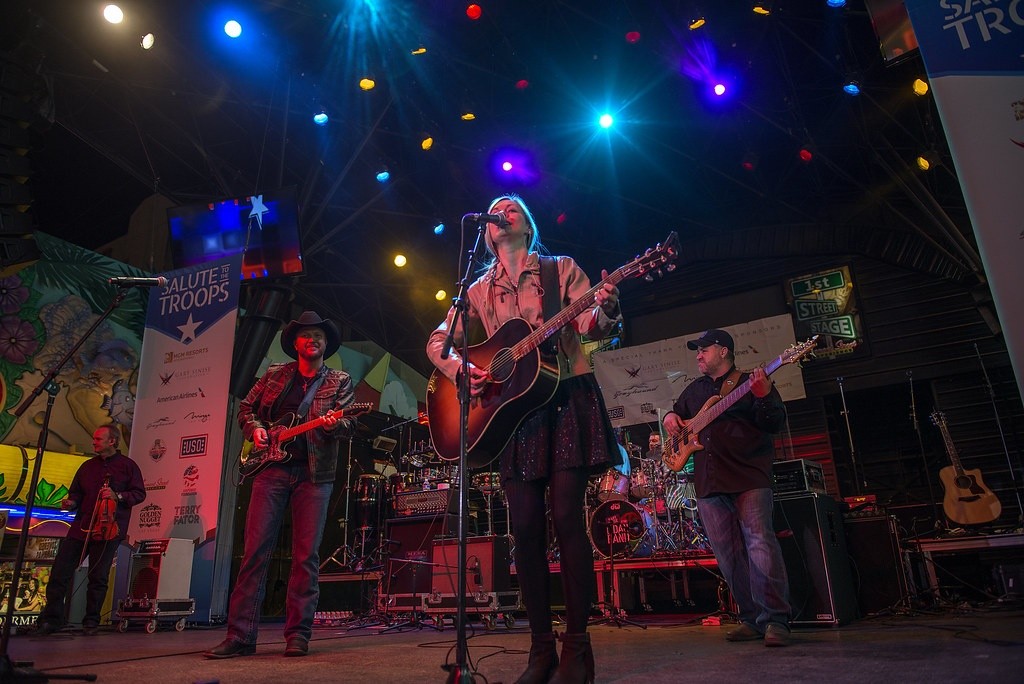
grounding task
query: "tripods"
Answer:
[344,558,474,634]
[318,437,359,570]
[624,462,713,560]
[685,561,738,624]
[586,525,647,630]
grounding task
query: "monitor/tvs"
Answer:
[165,184,307,287]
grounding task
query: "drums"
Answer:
[353,475,387,500]
[630,468,664,498]
[596,471,630,502]
[352,500,379,533]
[590,499,656,558]
[390,471,406,494]
[473,472,501,491]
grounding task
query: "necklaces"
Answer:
[303,374,315,391]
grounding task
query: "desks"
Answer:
[907,532,1024,603]
[604,554,740,621]
[318,571,386,582]
[510,560,606,615]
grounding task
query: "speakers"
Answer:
[772,492,861,625]
[383,513,471,607]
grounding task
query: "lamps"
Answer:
[422,213,446,235]
[751,0,778,16]
[686,5,706,30]
[842,68,867,95]
[917,149,942,171]
[912,74,932,98]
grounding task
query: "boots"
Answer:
[513,631,559,684]
[559,632,596,684]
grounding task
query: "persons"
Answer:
[203,311,357,657]
[662,327,791,645]
[427,196,624,684]
[16,425,146,635]
[649,433,662,449]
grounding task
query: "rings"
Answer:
[604,300,609,306]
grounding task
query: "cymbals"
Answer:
[646,446,662,461]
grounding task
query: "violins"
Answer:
[91,473,120,541]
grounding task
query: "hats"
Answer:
[280,311,342,361]
[686,329,735,351]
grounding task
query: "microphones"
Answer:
[474,557,481,584]
[469,212,506,228]
[108,276,168,287]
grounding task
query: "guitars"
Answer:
[238,402,374,479]
[929,406,1002,525]
[425,230,686,469]
[662,334,819,472]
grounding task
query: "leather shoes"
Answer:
[725,624,765,641]
[765,623,790,647]
[204,637,256,659]
[283,638,308,657]
[38,621,55,636]
[83,621,98,635]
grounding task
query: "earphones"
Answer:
[527,229,533,235]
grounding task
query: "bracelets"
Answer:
[116,491,123,500]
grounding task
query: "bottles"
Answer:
[140,593,149,609]
[423,478,430,490]
[124,593,132,607]
